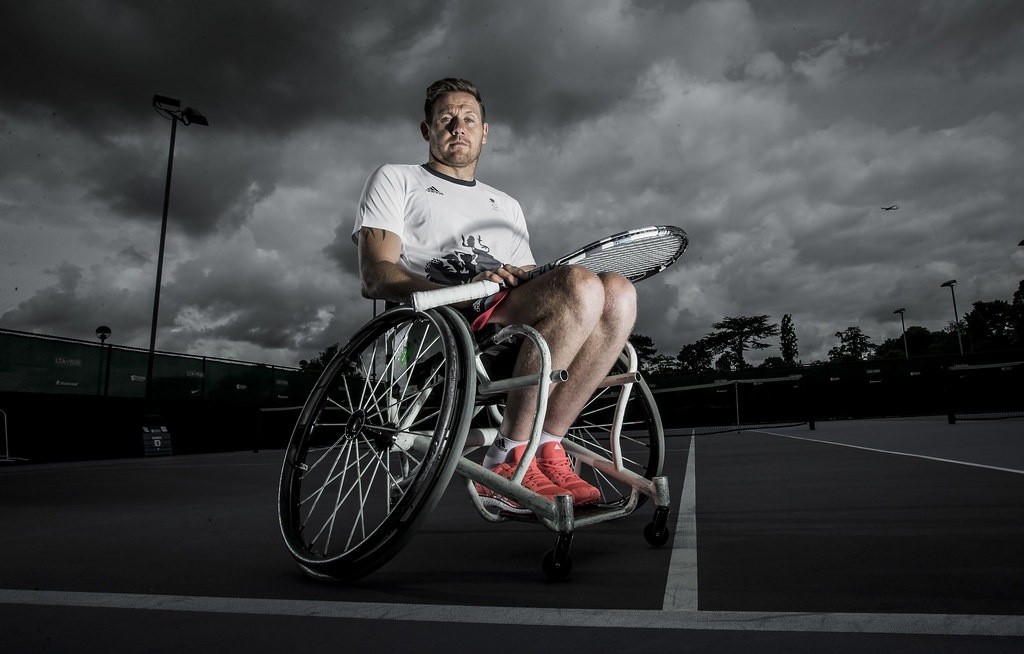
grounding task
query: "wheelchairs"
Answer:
[277,286,673,589]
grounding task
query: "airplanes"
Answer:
[880,204,900,212]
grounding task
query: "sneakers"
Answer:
[475,441,600,514]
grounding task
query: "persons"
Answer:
[351,76,636,515]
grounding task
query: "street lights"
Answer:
[94,322,112,397]
[939,275,972,356]
[140,88,208,416]
[893,304,912,357]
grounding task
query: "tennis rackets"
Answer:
[407,223,688,314]
